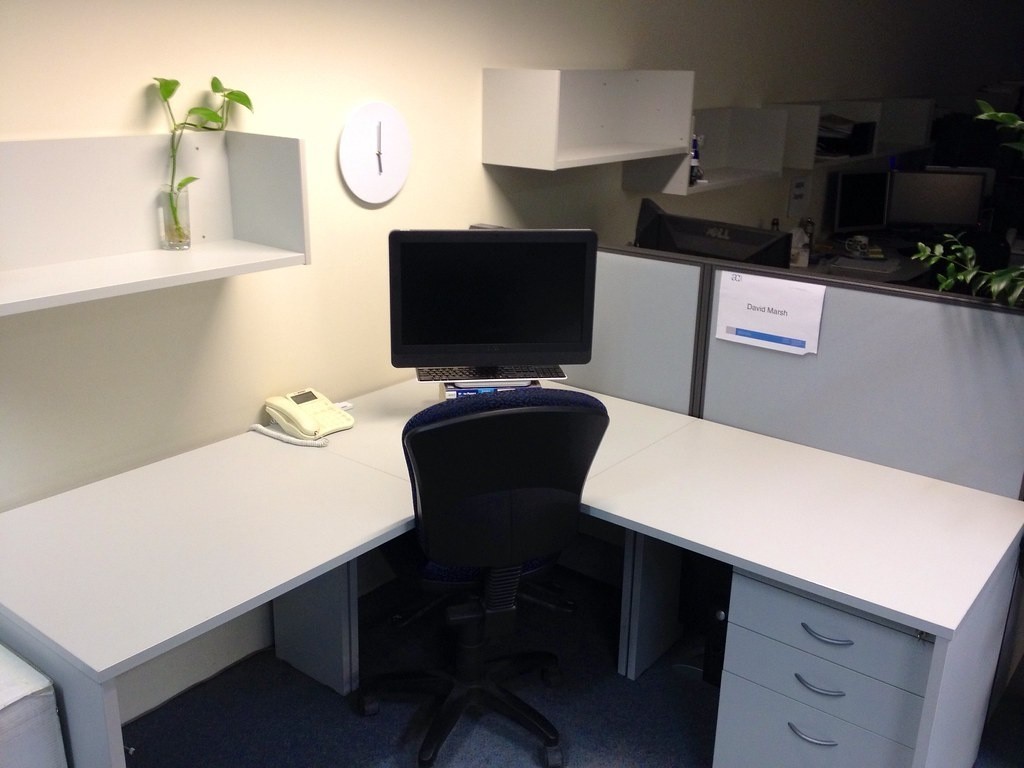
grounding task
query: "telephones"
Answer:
[264,387,354,441]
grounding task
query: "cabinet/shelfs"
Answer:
[481,66,933,195]
[0,130,313,315]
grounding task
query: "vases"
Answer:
[161,187,193,249]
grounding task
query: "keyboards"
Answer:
[416,365,567,381]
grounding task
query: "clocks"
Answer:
[338,100,414,206]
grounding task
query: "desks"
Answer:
[0,379,1024,768]
[809,227,981,284]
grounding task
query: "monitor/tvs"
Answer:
[887,170,985,241]
[828,170,892,235]
[632,197,792,270]
[388,229,597,388]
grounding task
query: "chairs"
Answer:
[930,113,1003,167]
[354,387,608,768]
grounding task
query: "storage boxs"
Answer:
[0,644,68,768]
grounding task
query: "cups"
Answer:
[846,235,868,258]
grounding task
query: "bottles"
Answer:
[689,135,700,185]
[771,219,780,231]
